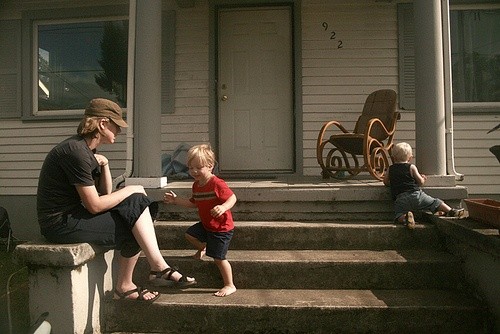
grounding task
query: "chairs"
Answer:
[316,89,401,181]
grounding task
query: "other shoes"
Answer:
[450,208,464,216]
[406,211,415,229]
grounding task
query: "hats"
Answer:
[85,98,128,128]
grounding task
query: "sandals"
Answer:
[148,268,198,287]
[112,288,161,304]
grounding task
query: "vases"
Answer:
[463,198,500,227]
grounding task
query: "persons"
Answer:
[37,99,196,304]
[383,142,464,229]
[164,144,236,297]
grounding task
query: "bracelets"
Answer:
[101,162,109,166]
